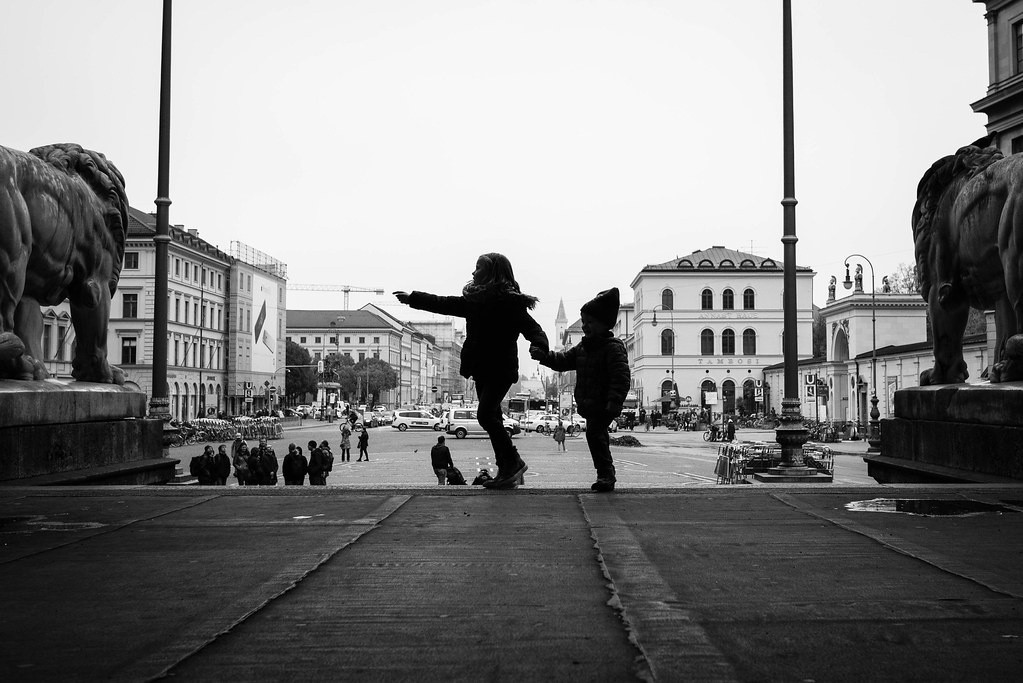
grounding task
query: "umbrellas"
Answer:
[653,395,685,402]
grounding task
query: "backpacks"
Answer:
[315,449,333,472]
[189,454,203,477]
[323,448,334,466]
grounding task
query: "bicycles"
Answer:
[338,417,366,433]
[737,413,840,443]
[703,425,736,443]
[170,415,286,447]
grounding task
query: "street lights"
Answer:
[651,304,676,419]
[842,253,881,452]
[322,321,338,421]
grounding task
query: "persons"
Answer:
[198,445,231,485]
[257,408,279,417]
[553,422,566,451]
[529,287,630,491]
[197,409,204,418]
[303,405,317,419]
[393,253,549,487]
[357,428,369,462]
[346,404,358,432]
[725,419,735,442]
[619,407,720,441]
[282,440,333,485]
[231,433,278,485]
[340,425,352,462]
[431,436,453,485]
[744,407,775,427]
[217,410,226,420]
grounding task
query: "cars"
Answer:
[510,397,618,437]
[410,393,480,418]
[346,404,395,428]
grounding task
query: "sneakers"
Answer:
[496,456,529,487]
[482,470,518,488]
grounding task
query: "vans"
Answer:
[392,410,442,432]
[446,407,522,438]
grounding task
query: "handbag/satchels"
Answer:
[339,442,344,447]
[356,439,361,449]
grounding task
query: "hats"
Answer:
[308,440,317,448]
[235,432,242,439]
[579,286,621,329]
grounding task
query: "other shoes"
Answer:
[356,458,361,461]
[364,458,369,461]
[591,470,617,492]
